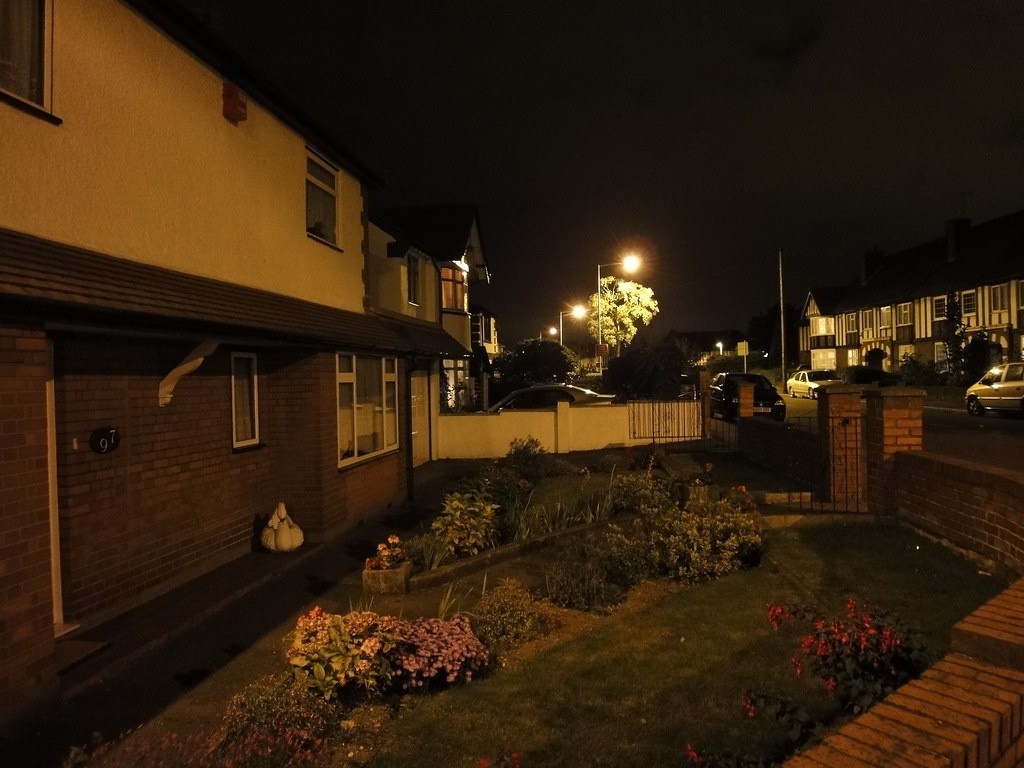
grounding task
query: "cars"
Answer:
[710,372,787,422]
[477,384,618,406]
[795,364,811,372]
[964,362,1024,417]
[788,369,842,400]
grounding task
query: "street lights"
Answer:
[596,256,641,372]
[559,306,587,344]
